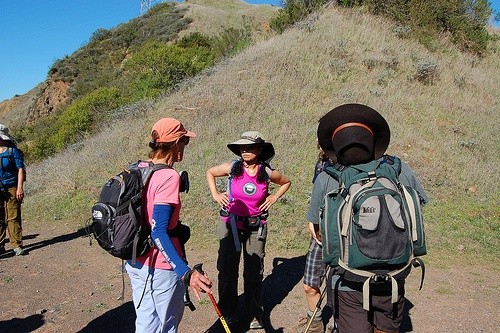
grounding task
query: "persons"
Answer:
[0,124,27,256]
[124,117,213,333]
[307,103,429,333]
[303,142,337,333]
[206,130,291,333]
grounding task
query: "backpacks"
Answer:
[84,159,175,266]
[319,154,427,311]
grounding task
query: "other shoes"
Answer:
[249,317,263,329]
[13,246,25,255]
[219,316,232,329]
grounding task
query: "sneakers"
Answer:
[289,314,325,333]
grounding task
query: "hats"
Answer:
[0,123,13,140]
[227,131,275,161]
[317,103,390,168]
[151,118,196,142]
